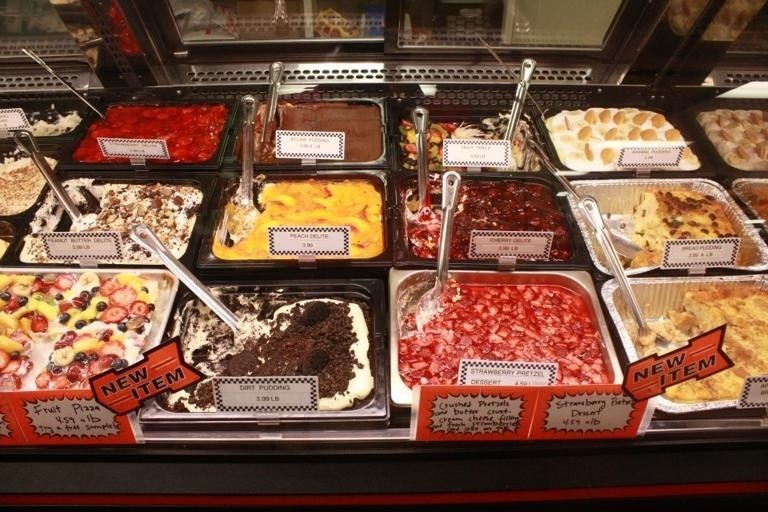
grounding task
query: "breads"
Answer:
[565,110,768,404]
